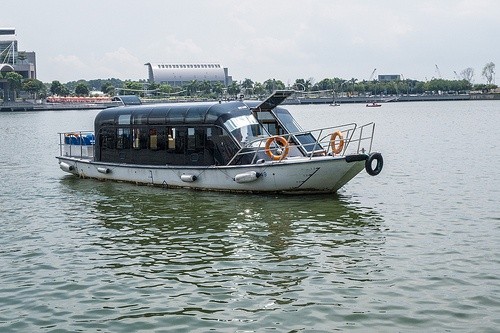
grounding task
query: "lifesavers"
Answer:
[330,132,344,154]
[266,136,289,160]
[365,152,384,176]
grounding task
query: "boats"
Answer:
[366,101,382,107]
[329,101,340,106]
[54,88,384,198]
